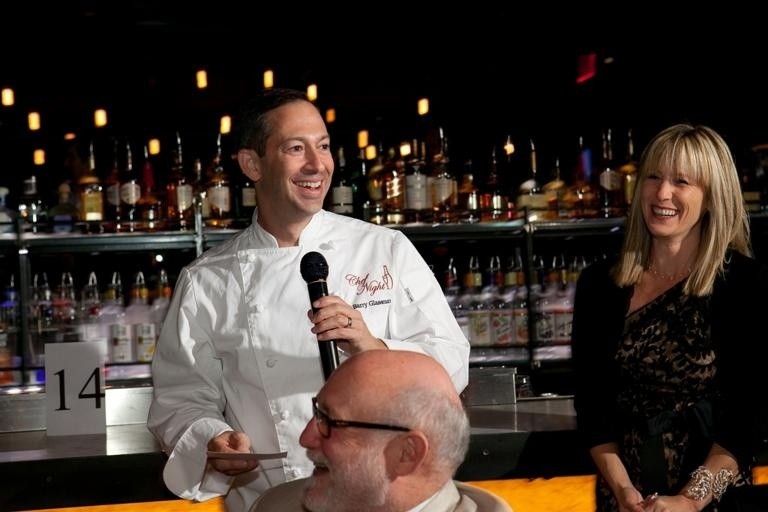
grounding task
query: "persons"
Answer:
[146,87,471,512]
[567,122,767,512]
[247,349,512,512]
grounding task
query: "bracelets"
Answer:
[686,465,734,501]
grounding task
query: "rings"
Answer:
[345,316,353,328]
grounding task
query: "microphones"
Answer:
[300,252,339,382]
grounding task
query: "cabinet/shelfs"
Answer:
[0,209,767,436]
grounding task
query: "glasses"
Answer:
[311,396,411,439]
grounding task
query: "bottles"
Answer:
[0,124,260,235]
[0,268,174,368]
[332,129,640,222]
[437,251,587,348]
